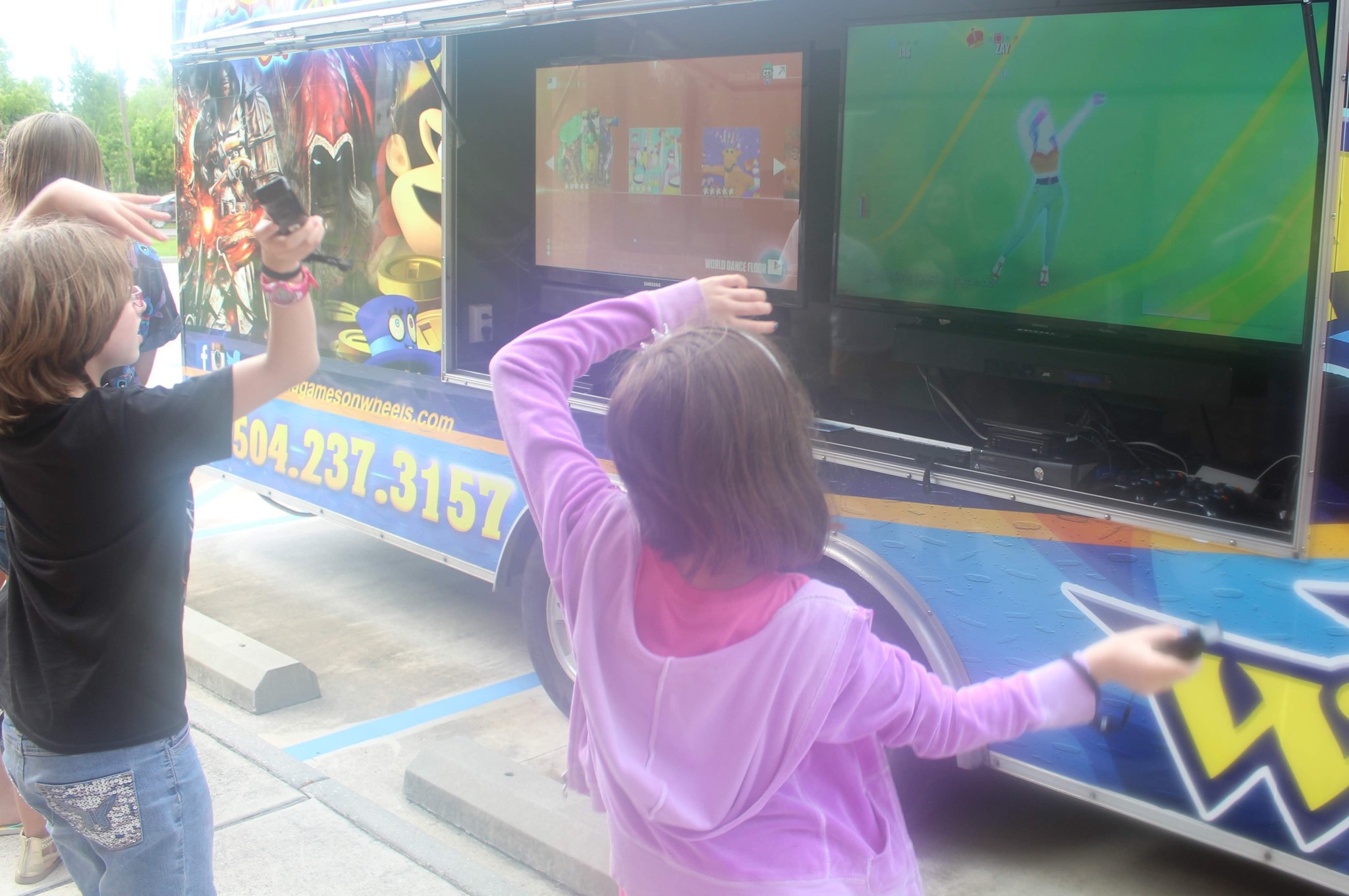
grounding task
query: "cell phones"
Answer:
[1161,624,1220,658]
[255,176,310,237]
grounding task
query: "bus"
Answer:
[172,1,1349,896]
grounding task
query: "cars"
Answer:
[138,193,176,229]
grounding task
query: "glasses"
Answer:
[126,285,143,307]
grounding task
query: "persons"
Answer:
[489,275,1200,896]
[0,176,325,896]
[0,113,181,883]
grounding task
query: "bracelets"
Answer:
[260,261,302,280]
[1063,652,1122,732]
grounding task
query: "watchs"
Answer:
[258,266,319,306]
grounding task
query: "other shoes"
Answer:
[0,822,23,836]
[16,828,62,885]
